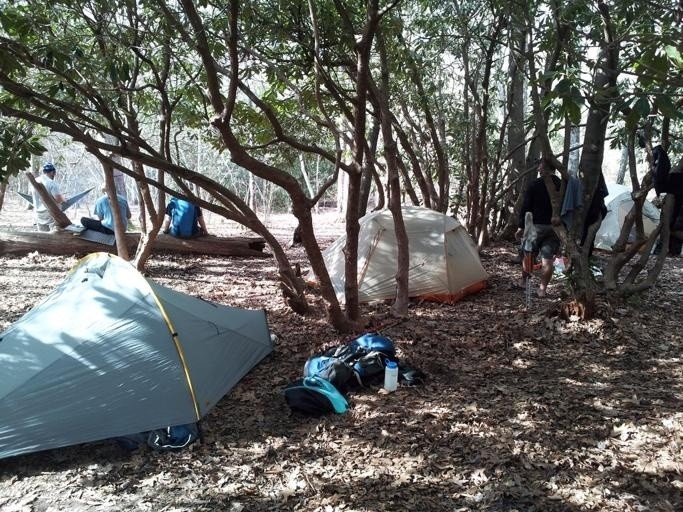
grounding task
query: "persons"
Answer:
[26,161,65,233]
[161,188,210,240]
[79,180,132,236]
[514,156,564,299]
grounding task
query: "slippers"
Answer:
[510,281,527,291]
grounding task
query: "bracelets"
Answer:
[162,230,169,234]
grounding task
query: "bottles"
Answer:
[384,357,398,392]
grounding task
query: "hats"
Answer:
[42,162,54,171]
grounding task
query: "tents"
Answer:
[0,251,275,461]
[304,203,492,307]
[591,184,662,255]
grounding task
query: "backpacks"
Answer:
[302,332,396,390]
